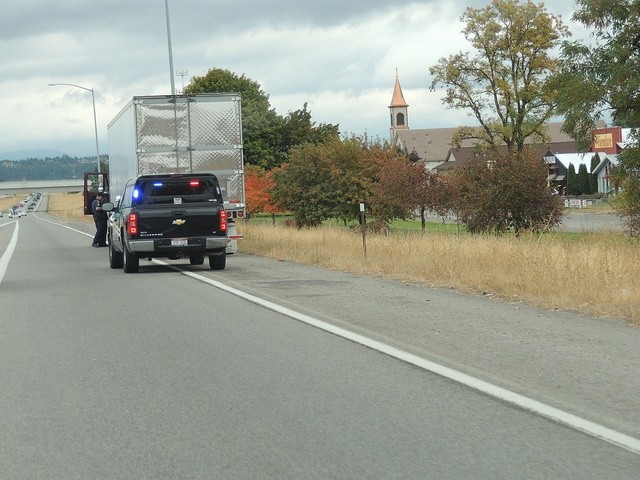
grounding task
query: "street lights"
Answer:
[46,80,105,183]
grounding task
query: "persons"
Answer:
[90,193,110,249]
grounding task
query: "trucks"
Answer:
[83,91,246,254]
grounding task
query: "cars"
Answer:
[0,192,41,218]
[99,172,228,273]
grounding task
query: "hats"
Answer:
[98,192,106,196]
